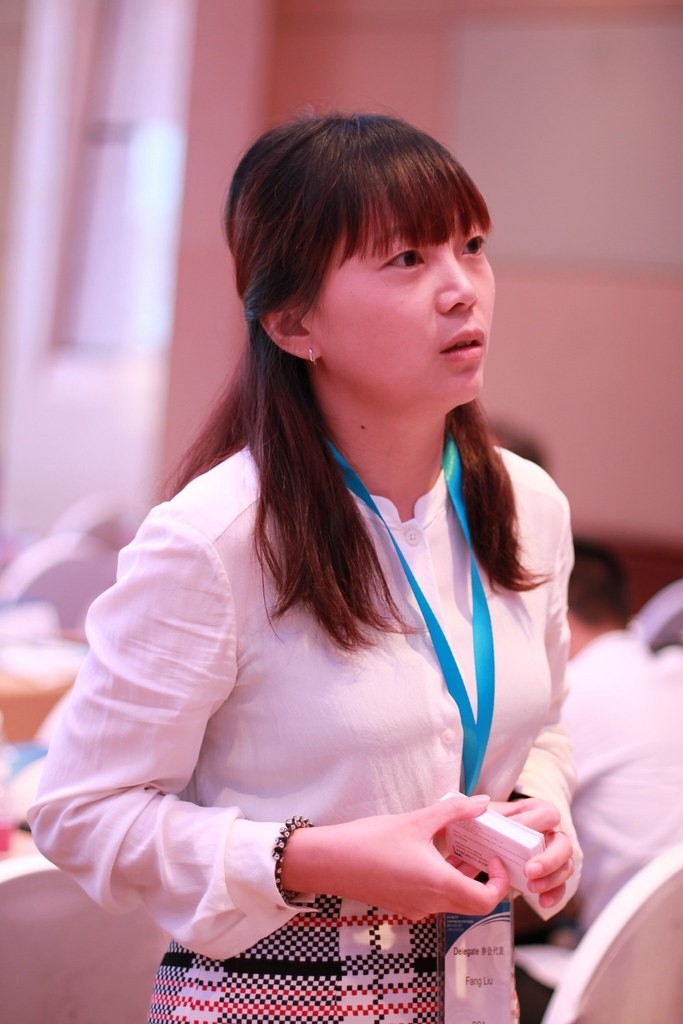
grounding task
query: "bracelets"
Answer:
[273,816,314,902]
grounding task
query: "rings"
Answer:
[571,857,574,864]
[547,831,567,841]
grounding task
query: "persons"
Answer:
[29,114,586,1023]
[514,538,682,1023]
